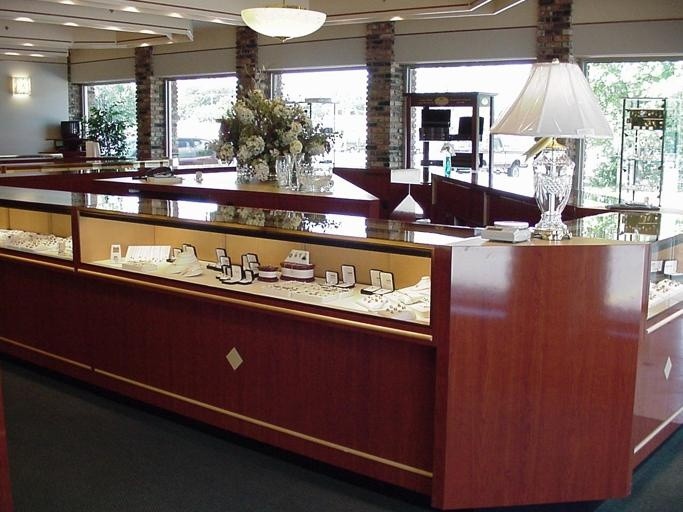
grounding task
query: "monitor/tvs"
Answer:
[61,121,80,137]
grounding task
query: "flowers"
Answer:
[216,64,344,183]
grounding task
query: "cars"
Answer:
[175,137,218,166]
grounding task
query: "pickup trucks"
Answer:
[451,133,529,179]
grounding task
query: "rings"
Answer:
[9,230,57,249]
[649,279,680,301]
[273,280,405,316]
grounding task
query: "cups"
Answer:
[273,150,334,194]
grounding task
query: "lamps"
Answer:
[488,61,614,243]
[8,76,33,95]
[240,0,327,44]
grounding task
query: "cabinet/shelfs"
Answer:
[429,173,577,227]
[405,91,498,227]
[37,138,94,155]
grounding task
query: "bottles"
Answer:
[443,147,451,175]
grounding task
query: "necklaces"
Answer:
[128,245,165,263]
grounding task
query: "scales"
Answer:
[480,221,531,243]
